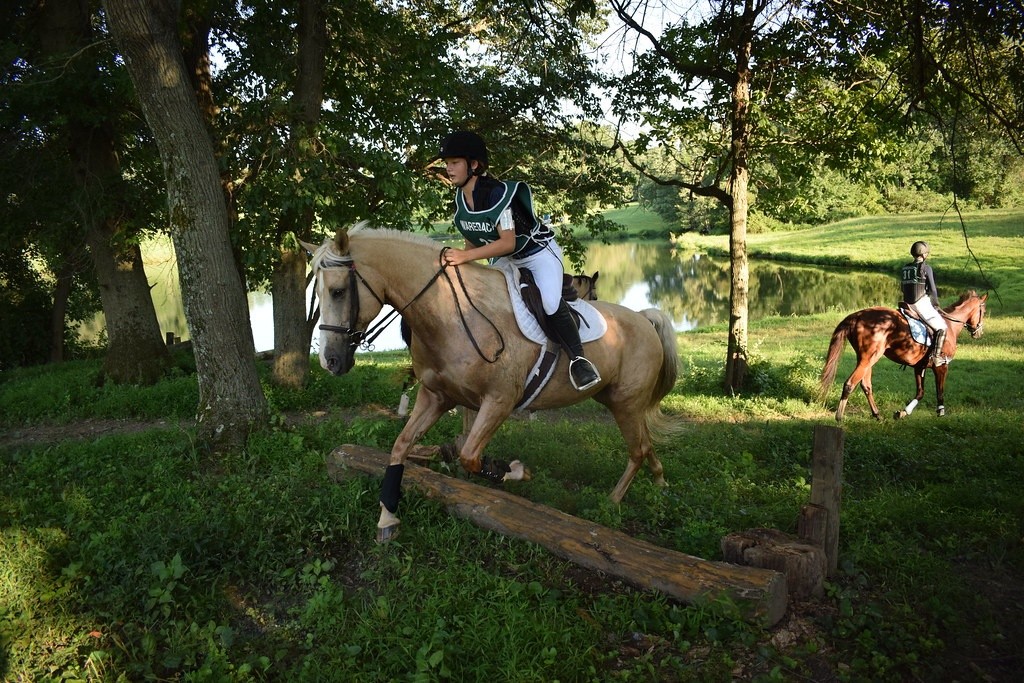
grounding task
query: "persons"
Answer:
[901,241,947,367]
[440,131,598,388]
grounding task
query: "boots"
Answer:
[546,297,596,387]
[933,329,952,367]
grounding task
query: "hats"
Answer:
[432,131,488,169]
[911,241,929,255]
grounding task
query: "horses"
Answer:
[296,222,678,545]
[820,289,989,422]
